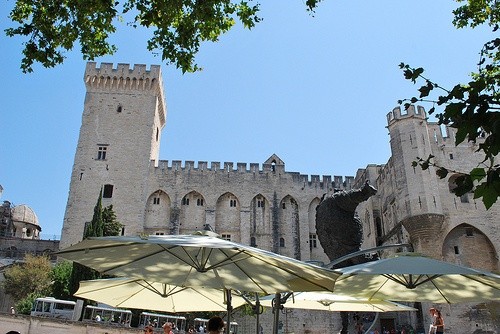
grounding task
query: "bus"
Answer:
[190,318,238,334]
[81,304,132,328]
[139,312,188,334]
[32,297,77,319]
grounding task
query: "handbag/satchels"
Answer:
[428,325,437,334]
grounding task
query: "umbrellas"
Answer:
[53,229,342,334]
[240,290,419,311]
[257,257,500,303]
[73,277,250,313]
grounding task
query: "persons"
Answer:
[428,307,438,334]
[92,314,226,334]
[356,323,425,334]
[434,310,445,334]
